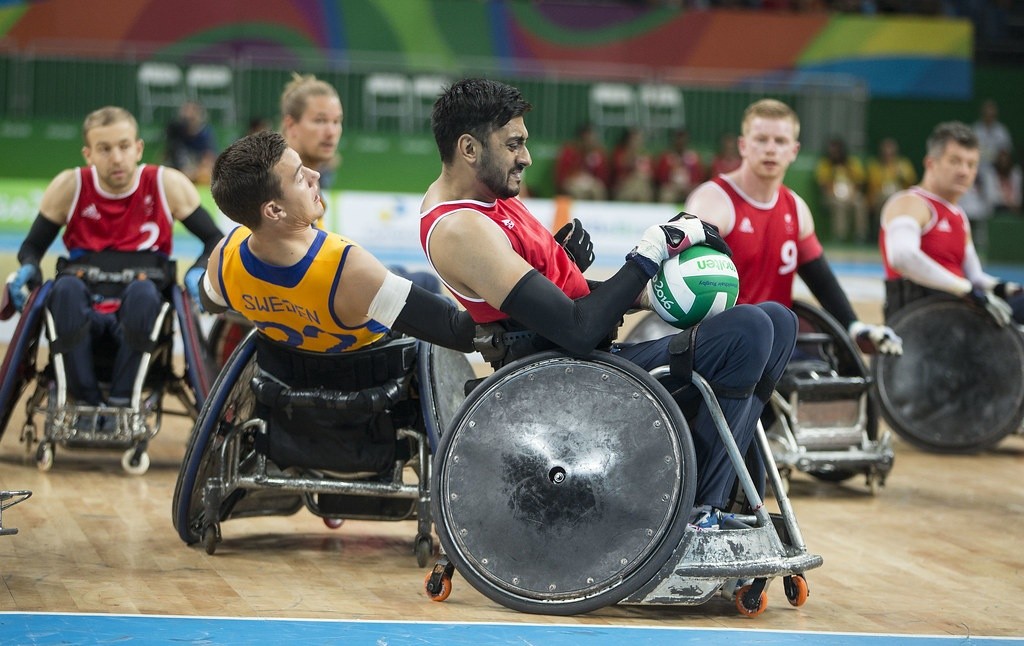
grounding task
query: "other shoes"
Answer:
[693,509,725,530]
[74,398,130,432]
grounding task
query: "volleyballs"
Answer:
[644,245,741,330]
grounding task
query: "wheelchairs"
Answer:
[0,277,211,478]
[428,347,823,618]
[175,325,478,569]
[872,287,1024,452]
[766,297,905,498]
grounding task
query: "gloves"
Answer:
[8,257,43,313]
[995,281,1020,301]
[185,256,214,315]
[553,218,595,273]
[626,212,733,278]
[849,321,903,357]
[967,283,1012,328]
[640,287,654,311]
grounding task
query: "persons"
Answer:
[417,77,801,533]
[167,103,215,193]
[558,98,1023,245]
[683,99,907,357]
[199,127,597,356]
[876,122,1023,331]
[272,72,448,301]
[9,103,229,408]
